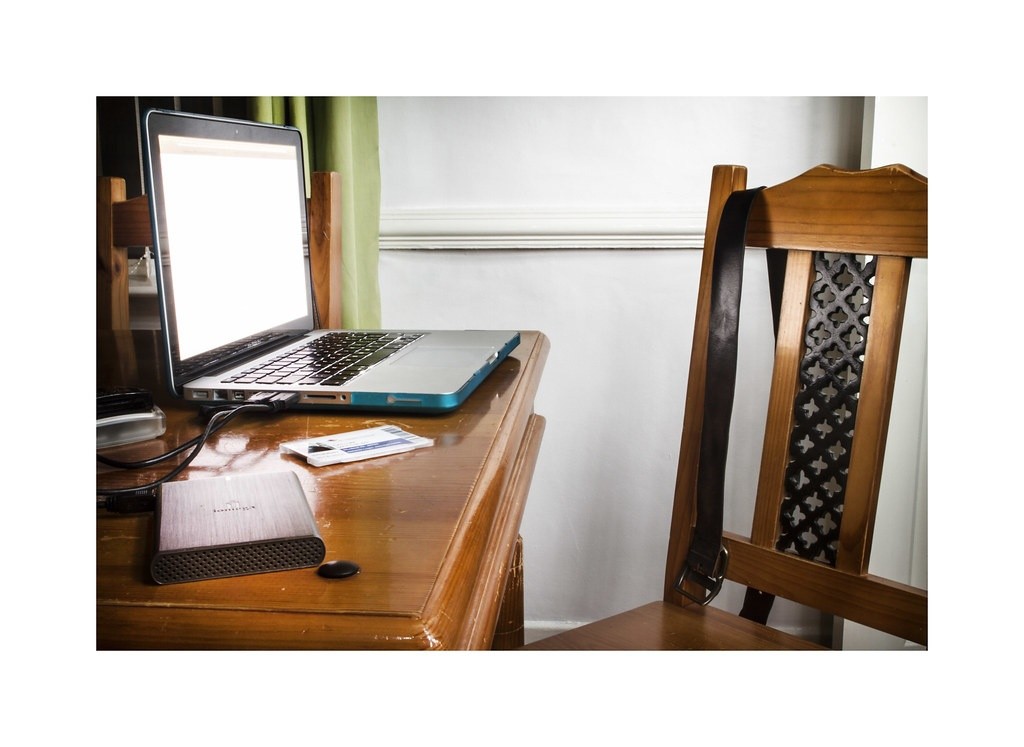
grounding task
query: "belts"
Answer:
[668,183,789,626]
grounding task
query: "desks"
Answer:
[96,329,551,651]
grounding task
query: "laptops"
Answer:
[142,109,521,412]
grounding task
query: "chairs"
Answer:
[96,171,342,330]
[507,163,928,651]
